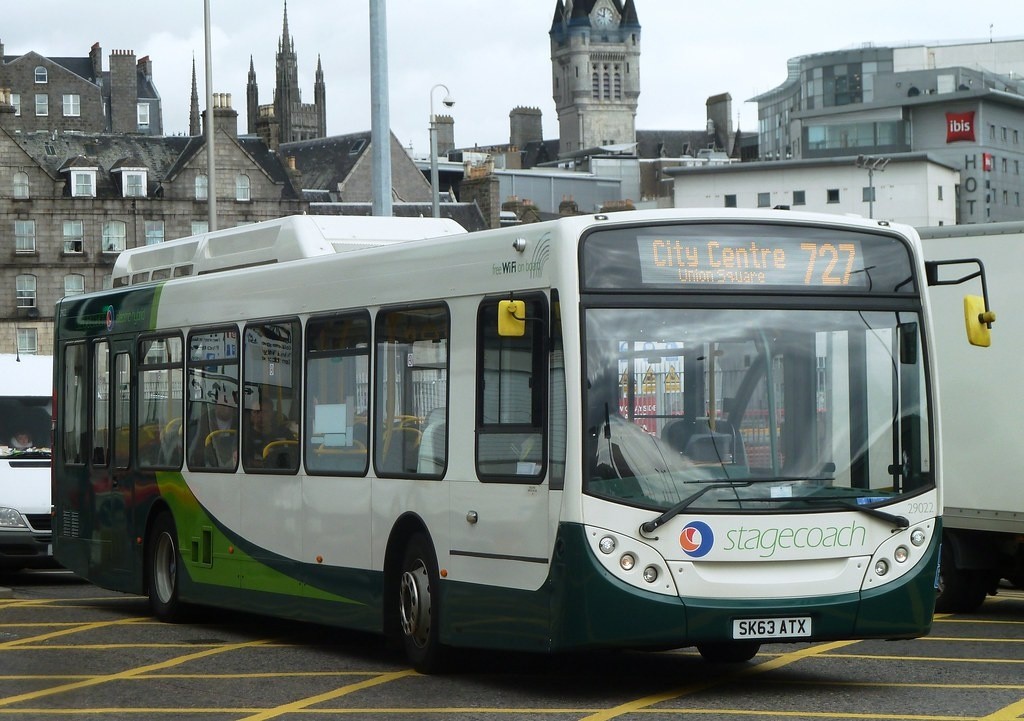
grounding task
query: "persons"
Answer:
[183,386,302,470]
[8,431,33,451]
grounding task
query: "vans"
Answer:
[0,353,79,579]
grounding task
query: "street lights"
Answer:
[429,82,456,217]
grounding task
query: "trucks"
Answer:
[827,219,1024,611]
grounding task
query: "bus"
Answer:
[49,206,996,683]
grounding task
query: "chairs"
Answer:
[660,417,752,484]
[75,414,424,471]
[416,419,445,479]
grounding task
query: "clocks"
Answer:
[595,7,615,29]
